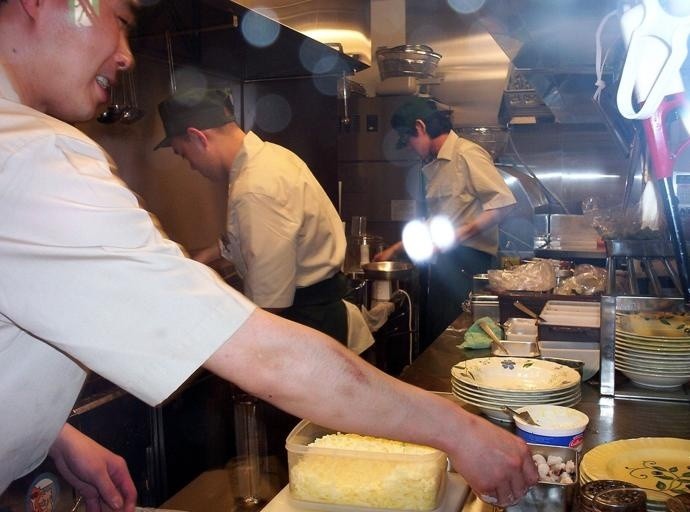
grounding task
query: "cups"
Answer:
[511,404,590,463]
[349,215,368,237]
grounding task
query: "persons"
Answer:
[151,84,377,357]
[0,0,538,512]
[373,99,517,339]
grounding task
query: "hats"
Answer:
[391,98,455,150]
[153,87,238,151]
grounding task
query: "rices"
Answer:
[290,431,449,512]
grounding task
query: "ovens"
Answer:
[336,90,453,247]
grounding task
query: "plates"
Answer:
[614,311,690,391]
[450,357,584,424]
[578,436,690,512]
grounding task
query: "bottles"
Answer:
[360,238,371,269]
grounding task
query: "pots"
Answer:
[360,261,415,281]
[374,76,445,95]
